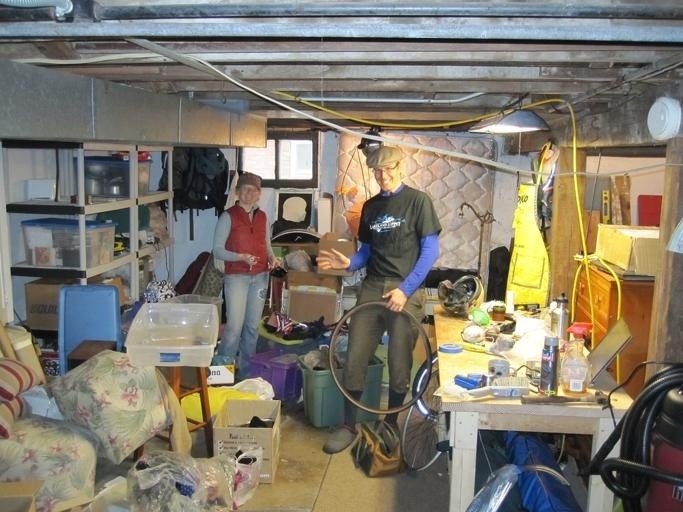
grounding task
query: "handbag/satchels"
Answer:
[353,421,402,477]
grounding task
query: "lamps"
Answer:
[495,110,550,134]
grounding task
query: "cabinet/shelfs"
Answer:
[573,256,655,401]
[0,141,174,339]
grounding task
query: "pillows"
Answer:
[0,356,43,438]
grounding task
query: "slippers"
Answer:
[323,425,358,453]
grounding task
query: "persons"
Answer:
[315,147,442,453]
[211,172,282,379]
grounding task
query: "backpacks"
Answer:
[155,146,228,210]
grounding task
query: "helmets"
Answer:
[438,275,484,317]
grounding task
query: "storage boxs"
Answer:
[596,223,658,275]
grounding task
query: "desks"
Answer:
[434,304,633,512]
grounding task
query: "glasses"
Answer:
[372,162,399,174]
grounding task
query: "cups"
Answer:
[487,359,517,386]
[493,305,506,321]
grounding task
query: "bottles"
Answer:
[540,334,560,396]
[560,339,591,397]
[551,292,572,343]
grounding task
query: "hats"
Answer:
[367,146,403,168]
[237,174,261,191]
